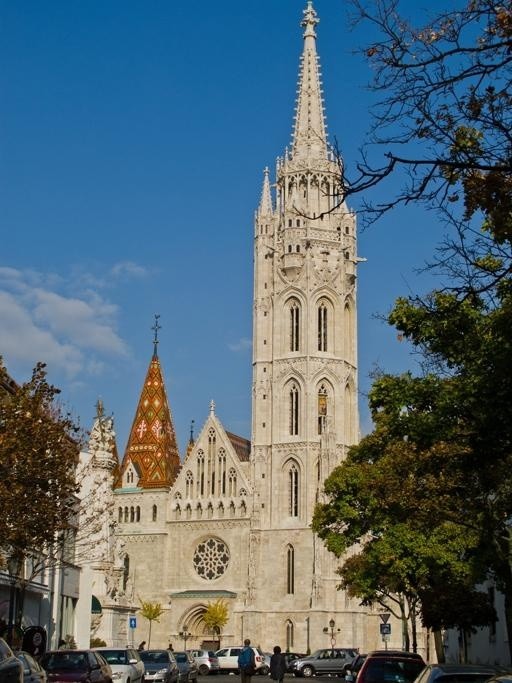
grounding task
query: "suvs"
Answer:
[289,648,361,678]
[215,645,266,675]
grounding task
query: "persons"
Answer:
[139,639,145,650]
[238,639,255,682]
[165,643,174,650]
[270,645,286,682]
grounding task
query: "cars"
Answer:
[282,653,310,672]
[172,651,199,683]
[414,665,498,683]
[346,654,367,683]
[485,673,511,683]
[89,648,145,683]
[188,649,222,676]
[38,650,114,682]
[1,638,25,683]
[261,651,274,674]
[346,650,428,683]
[140,649,179,683]
[13,650,48,683]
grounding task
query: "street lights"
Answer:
[6,548,25,646]
[323,618,341,649]
[179,625,194,650]
